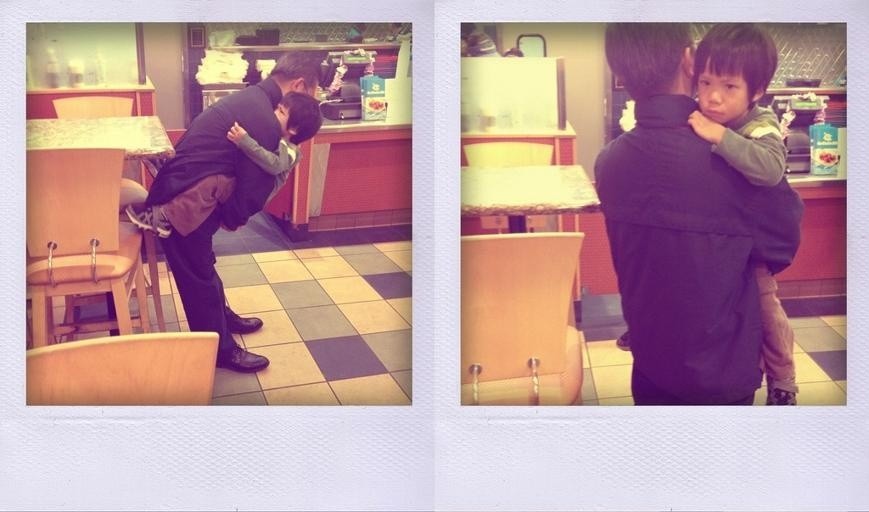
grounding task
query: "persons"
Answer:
[144,51,323,373]
[123,92,322,239]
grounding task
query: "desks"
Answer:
[461,158,603,238]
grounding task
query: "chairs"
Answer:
[461,140,587,406]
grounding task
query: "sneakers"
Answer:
[616,330,633,352]
[125,202,173,239]
[765,386,797,405]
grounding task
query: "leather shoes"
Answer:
[226,305,263,333]
[216,344,270,373]
